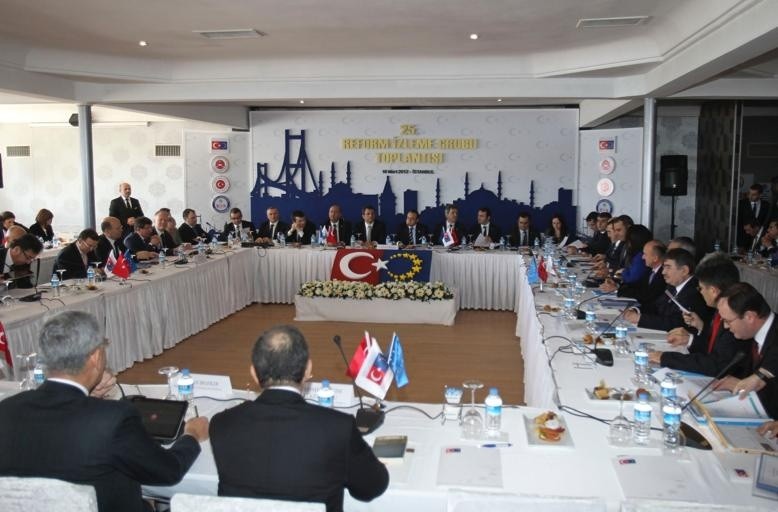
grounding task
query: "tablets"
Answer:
[131,396,188,441]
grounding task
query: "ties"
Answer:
[160,235,165,248]
[236,225,526,245]
[751,340,759,370]
[708,313,720,351]
[126,199,129,208]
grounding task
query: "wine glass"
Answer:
[461,377,484,438]
[17,352,37,391]
[1,234,221,311]
[158,366,178,401]
[608,386,632,446]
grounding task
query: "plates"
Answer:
[525,413,574,449]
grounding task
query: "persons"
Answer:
[86,208,216,268]
[220,208,505,250]
[109,182,142,244]
[579,212,778,440]
[208,324,389,512]
[736,183,770,252]
[0,207,55,293]
[1,310,210,510]
[510,212,578,249]
[50,228,100,284]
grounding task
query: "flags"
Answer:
[345,333,408,401]
[103,247,139,286]
[331,249,433,286]
[527,255,550,291]
[0,323,14,367]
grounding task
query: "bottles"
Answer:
[35,357,47,387]
[239,234,514,252]
[317,379,334,410]
[484,387,503,434]
[442,382,465,429]
[179,367,194,421]
[714,238,772,269]
[530,238,690,452]
[50,232,234,297]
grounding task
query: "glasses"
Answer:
[22,250,34,263]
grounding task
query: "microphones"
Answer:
[206,221,220,232]
[681,351,746,450]
[333,335,384,435]
[594,301,633,365]
[18,258,41,302]
[575,284,626,318]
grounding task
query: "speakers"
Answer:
[660,155,688,196]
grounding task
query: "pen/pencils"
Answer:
[194,406,199,417]
